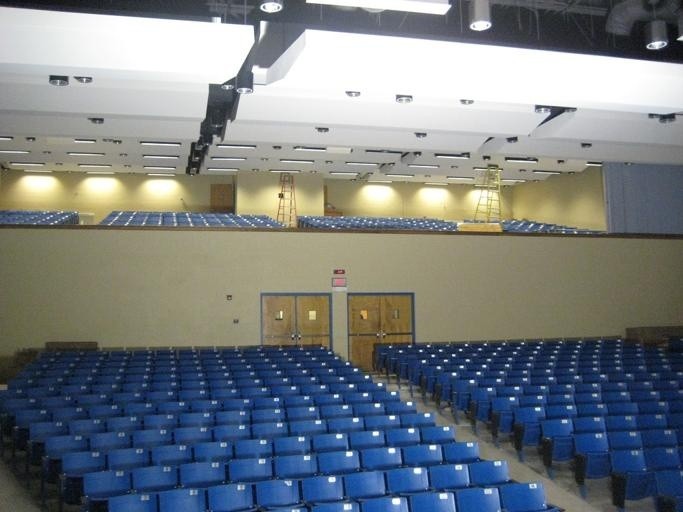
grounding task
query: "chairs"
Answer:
[99,212,288,229]
[299,214,458,232]
[1,211,81,228]
[370,339,682,507]
[4,341,563,510]
[464,217,609,237]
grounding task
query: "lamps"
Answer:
[644,0,683,51]
[395,95,413,104]
[185,0,285,176]
[660,113,676,124]
[535,105,552,114]
[468,0,492,31]
[49,76,69,88]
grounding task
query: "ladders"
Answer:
[474,164,502,226]
[276,175,297,226]
